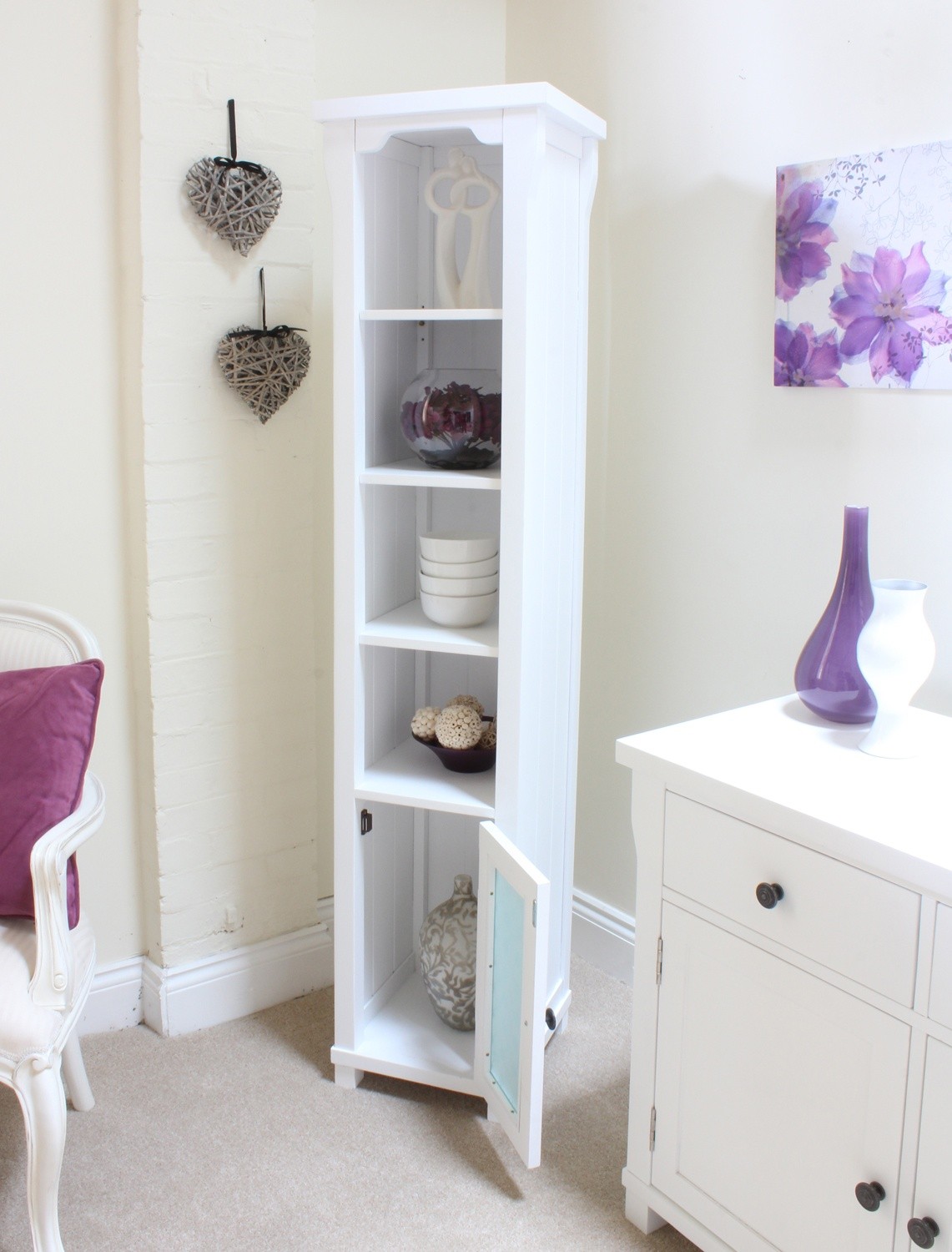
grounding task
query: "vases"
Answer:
[857,578,934,759]
[794,504,877,724]
[420,874,477,1031]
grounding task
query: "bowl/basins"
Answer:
[417,529,500,628]
[410,715,496,772]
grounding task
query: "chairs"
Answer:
[0,597,107,1252]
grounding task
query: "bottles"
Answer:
[398,367,501,469]
[419,874,476,1031]
[856,579,936,759]
[794,502,878,723]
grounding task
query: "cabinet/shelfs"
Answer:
[616,690,952,1252]
[312,83,607,1170]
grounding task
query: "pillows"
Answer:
[0,659,105,930]
